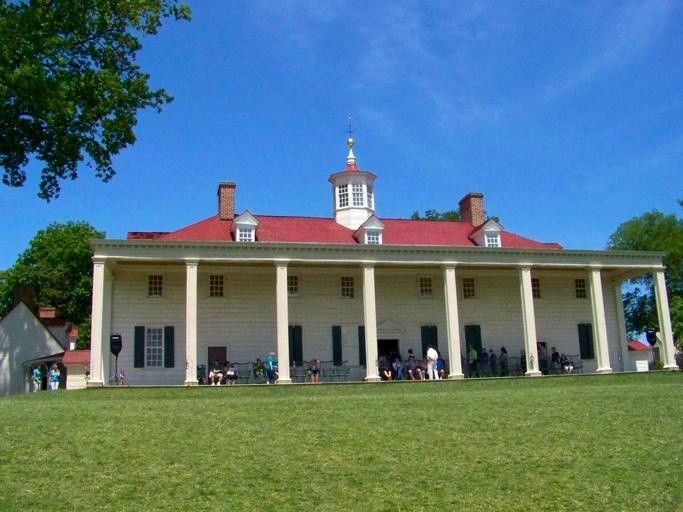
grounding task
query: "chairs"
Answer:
[204,358,351,385]
[377,350,585,382]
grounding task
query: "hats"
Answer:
[268,351,275,355]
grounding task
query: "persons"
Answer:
[262,350,278,384]
[30,366,42,392]
[253,354,264,383]
[224,361,238,385]
[209,359,224,386]
[309,359,319,383]
[119,367,125,385]
[378,342,575,381]
[46,363,61,390]
[288,360,297,384]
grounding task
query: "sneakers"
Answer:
[469,371,573,378]
[211,382,236,385]
[382,376,443,381]
